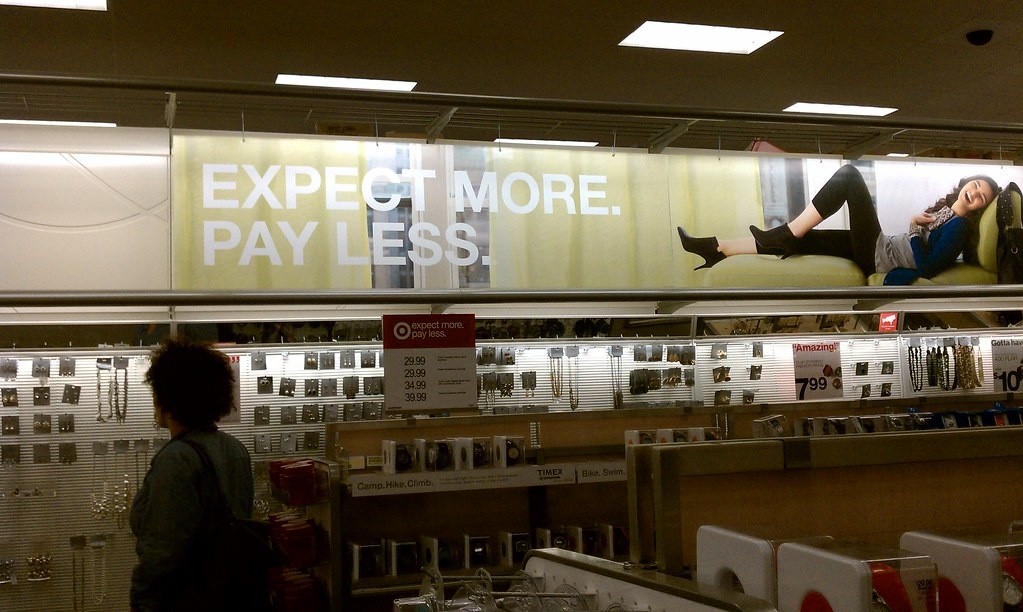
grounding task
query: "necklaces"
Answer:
[549,357,564,397]
[95,369,113,424]
[908,346,923,391]
[91,454,109,518]
[609,355,624,409]
[957,344,985,389]
[926,346,938,387]
[135,450,148,495]
[113,452,130,530]
[115,368,129,424]
[568,356,580,411]
[72,550,84,612]
[90,546,106,604]
[936,346,958,391]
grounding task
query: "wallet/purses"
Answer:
[925,205,954,232]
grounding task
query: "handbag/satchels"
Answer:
[996,182,1023,284]
[175,437,274,612]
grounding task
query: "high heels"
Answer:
[749,222,804,259]
[678,226,727,271]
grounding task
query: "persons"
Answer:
[126,337,254,612]
[676,163,998,279]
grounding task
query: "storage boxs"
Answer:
[493,435,526,467]
[348,538,386,579]
[1008,408,1023,425]
[751,414,792,437]
[388,522,631,612]
[625,430,657,448]
[416,438,458,474]
[690,427,722,440]
[820,416,850,434]
[935,411,963,428]
[906,411,938,430]
[457,436,492,470]
[877,413,914,432]
[959,411,988,427]
[986,408,1014,426]
[850,414,883,433]
[657,428,690,443]
[800,416,829,435]
[381,439,418,476]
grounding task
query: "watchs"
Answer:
[705,430,717,441]
[396,445,413,472]
[673,432,686,442]
[506,440,521,464]
[357,540,491,579]
[551,532,567,549]
[639,432,652,444]
[944,413,1023,430]
[473,442,485,468]
[767,418,877,436]
[513,535,532,563]
[437,442,451,470]
[889,416,905,431]
[916,415,930,430]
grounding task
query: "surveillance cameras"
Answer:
[966,29,994,47]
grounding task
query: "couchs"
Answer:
[704,188,1021,289]
